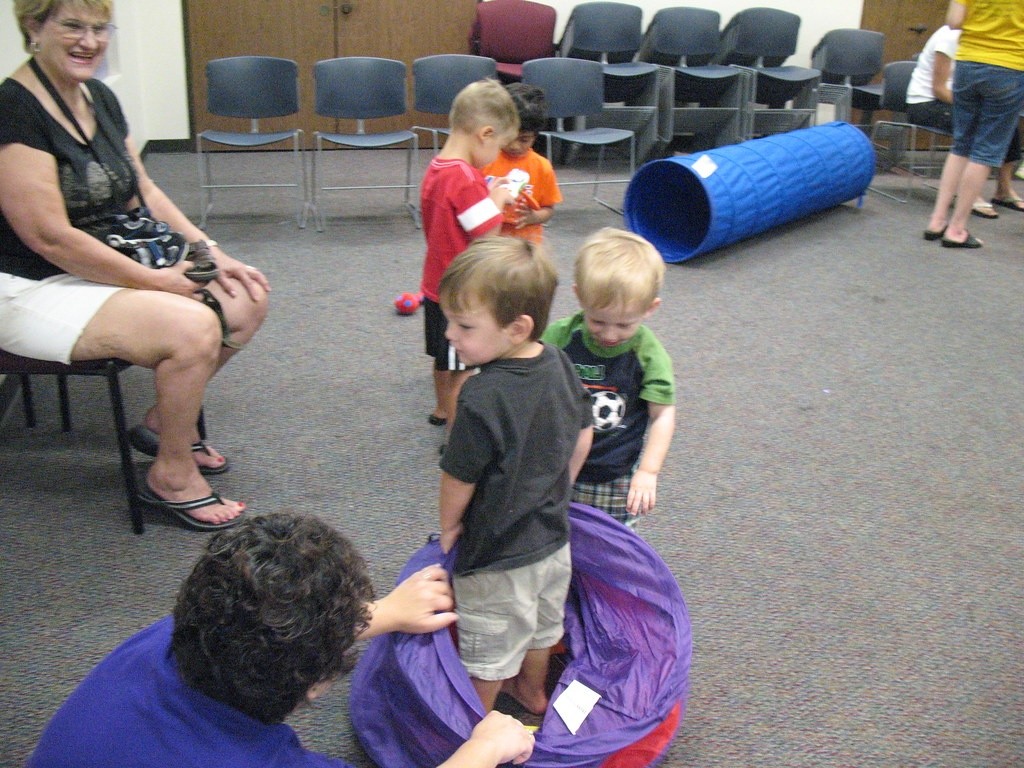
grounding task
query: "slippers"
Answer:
[127,425,228,473]
[991,197,1024,211]
[428,413,449,425]
[952,199,998,217]
[134,478,244,528]
[925,224,950,240]
[941,229,983,248]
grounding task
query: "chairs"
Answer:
[311,56,423,232]
[197,54,308,231]
[413,54,497,213]
[470,0,955,227]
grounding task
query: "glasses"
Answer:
[45,17,119,42]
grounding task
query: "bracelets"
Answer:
[206,240,219,247]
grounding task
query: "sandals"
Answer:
[186,239,218,279]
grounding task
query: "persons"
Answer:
[420,78,524,453]
[26,511,535,768]
[538,228,678,531]
[0,0,272,531]
[906,24,1024,219]
[478,83,563,244]
[924,0,1024,249]
[438,237,595,718]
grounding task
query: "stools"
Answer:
[0,347,206,534]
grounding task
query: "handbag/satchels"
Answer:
[107,214,186,272]
